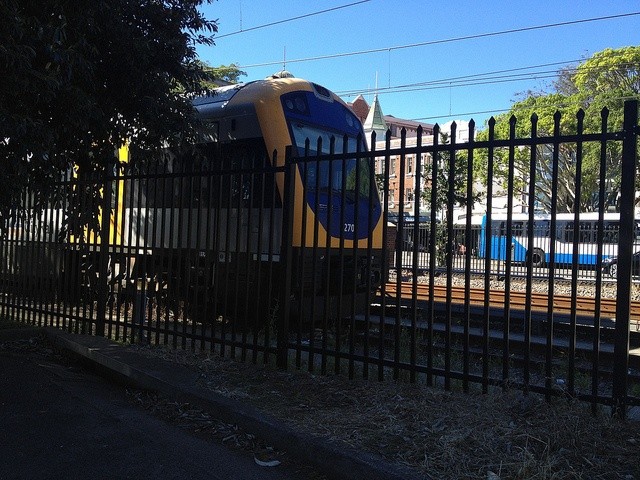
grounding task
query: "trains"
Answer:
[0,78,383,326]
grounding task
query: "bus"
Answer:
[479,214,640,278]
